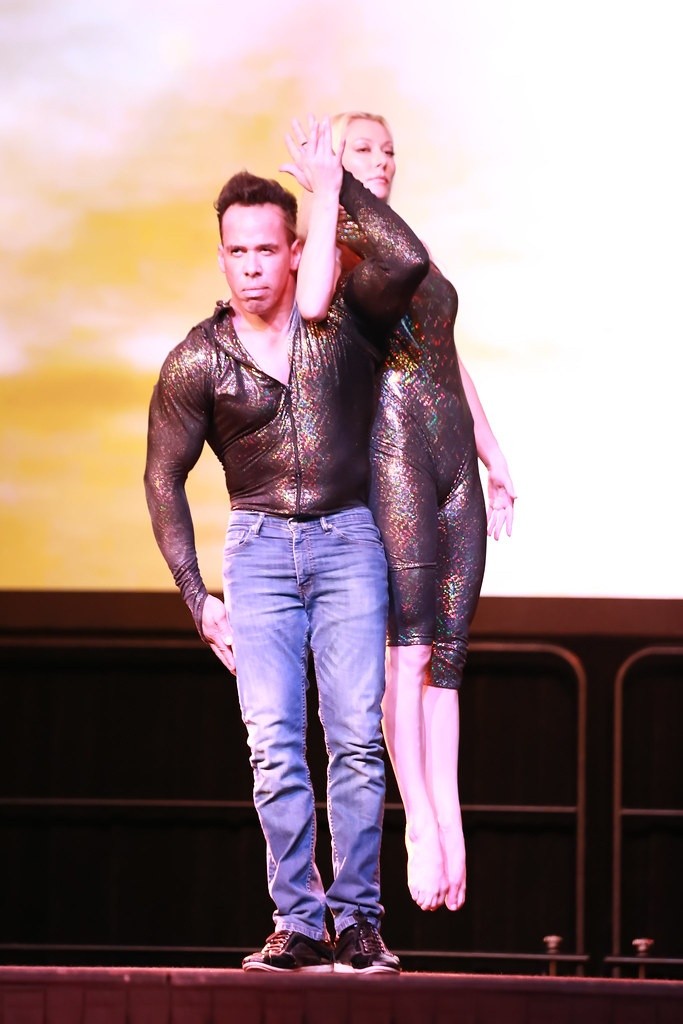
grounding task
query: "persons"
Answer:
[144,113,432,975]
[294,112,518,910]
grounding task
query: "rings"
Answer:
[302,142,307,146]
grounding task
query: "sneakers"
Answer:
[242,930,335,973]
[334,902,402,974]
